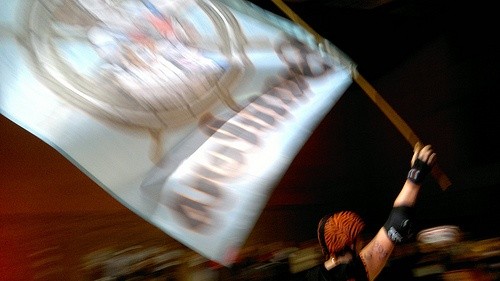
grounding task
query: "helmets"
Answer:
[319,209,363,257]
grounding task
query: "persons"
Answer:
[300,145,437,281]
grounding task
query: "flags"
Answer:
[0,0,354,268]
